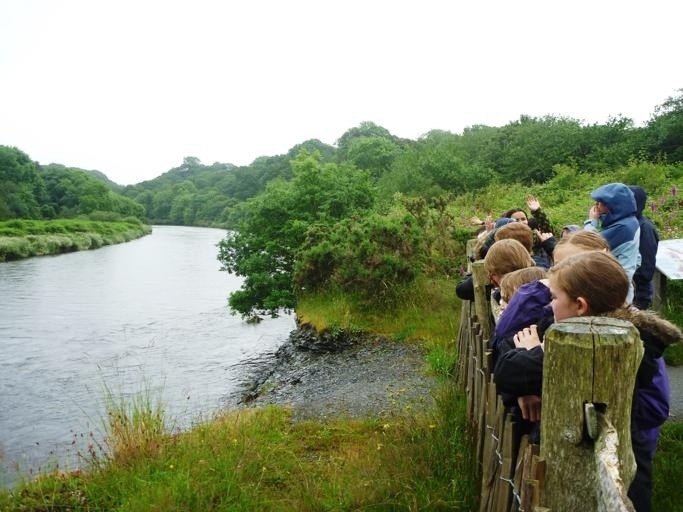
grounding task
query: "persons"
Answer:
[627,186,659,311]
[584,182,642,305]
[492,251,683,512]
[454,193,610,347]
